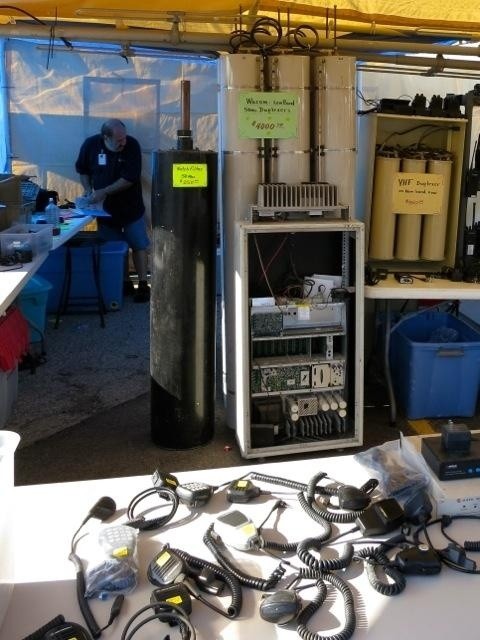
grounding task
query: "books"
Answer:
[71,195,112,219]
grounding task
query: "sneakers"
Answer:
[123,281,134,294]
[133,286,150,303]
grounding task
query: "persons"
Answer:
[76,118,150,303]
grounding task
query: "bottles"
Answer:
[45,197,60,236]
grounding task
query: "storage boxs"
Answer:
[37,232,128,312]
[375,310,480,420]
